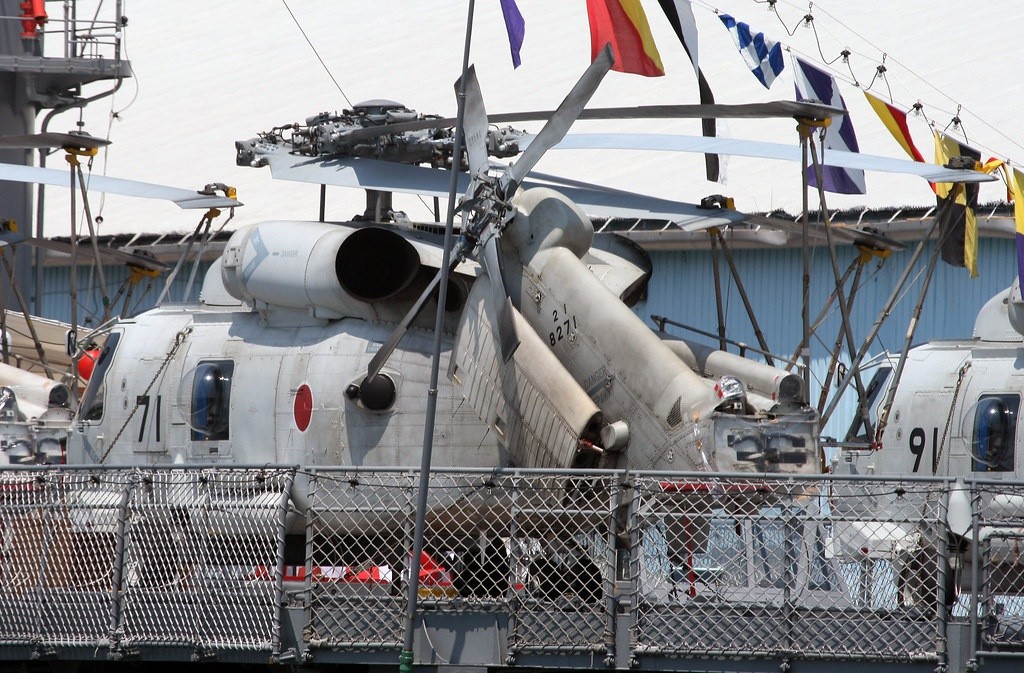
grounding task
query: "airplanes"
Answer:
[0,38,1024,609]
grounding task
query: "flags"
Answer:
[586,0,1024,300]
[500,0,525,70]
